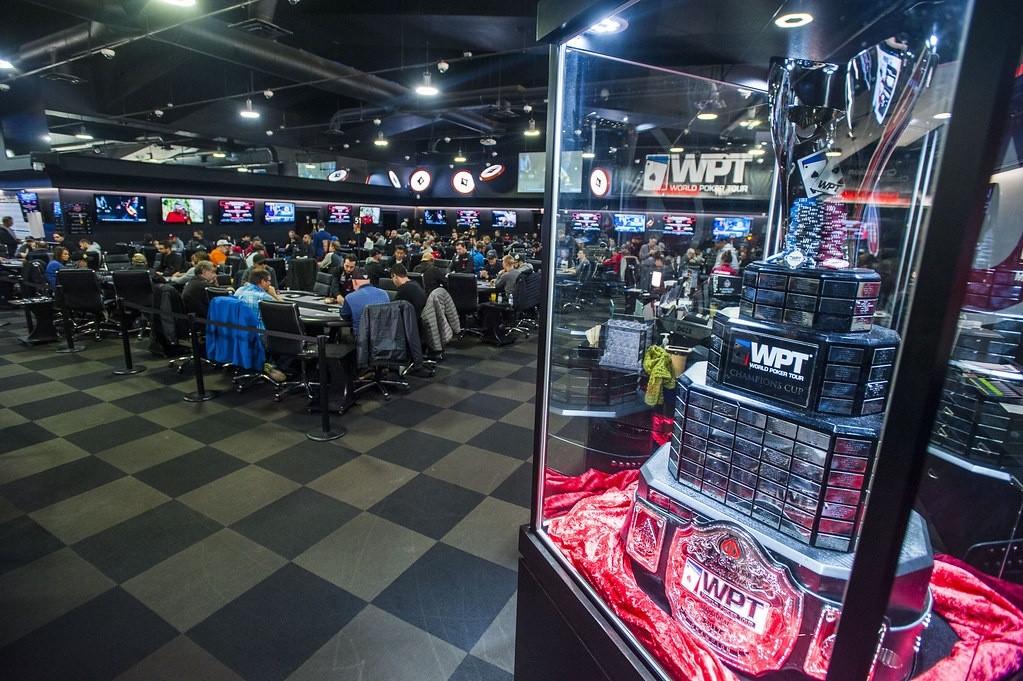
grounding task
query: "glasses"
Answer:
[265,279,273,284]
[258,262,266,265]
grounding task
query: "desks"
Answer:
[555,267,576,277]
[477,280,497,292]
[279,293,343,326]
[1,258,26,269]
[916,447,1023,587]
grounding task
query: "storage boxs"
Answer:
[596,314,657,372]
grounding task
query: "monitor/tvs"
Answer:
[160,197,205,224]
[456,210,481,226]
[661,215,696,237]
[571,212,601,232]
[424,209,448,225]
[359,206,381,225]
[94,194,148,224]
[491,210,517,229]
[327,204,352,224]
[613,213,646,233]
[712,217,754,246]
[264,201,296,223]
[219,200,254,224]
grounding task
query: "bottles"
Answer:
[498,293,503,303]
[508,294,513,306]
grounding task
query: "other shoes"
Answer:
[351,370,375,383]
[377,366,389,379]
[264,363,286,381]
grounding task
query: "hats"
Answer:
[351,268,370,279]
[254,236,261,240]
[711,234,728,242]
[253,254,265,263]
[487,249,497,259]
[217,239,231,246]
[169,234,175,240]
[24,235,35,242]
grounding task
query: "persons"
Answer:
[0,217,21,262]
[556,231,591,295]
[266,205,274,219]
[165,201,191,223]
[142,229,286,383]
[414,229,540,327]
[598,234,705,298]
[711,250,739,275]
[17,236,37,258]
[46,247,69,291]
[52,231,76,252]
[856,248,898,301]
[72,239,116,300]
[711,234,761,272]
[285,220,429,379]
[26,240,49,263]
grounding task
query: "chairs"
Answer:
[0,240,627,417]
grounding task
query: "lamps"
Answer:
[415,40,439,96]
[373,129,389,146]
[454,146,467,162]
[75,125,94,140]
[237,160,248,173]
[238,83,260,119]
[697,104,720,120]
[667,145,688,154]
[825,149,841,157]
[305,159,316,169]
[212,137,226,158]
[143,143,158,162]
[739,107,763,130]
[584,15,630,36]
[539,111,541,133]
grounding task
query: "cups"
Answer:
[491,292,497,303]
